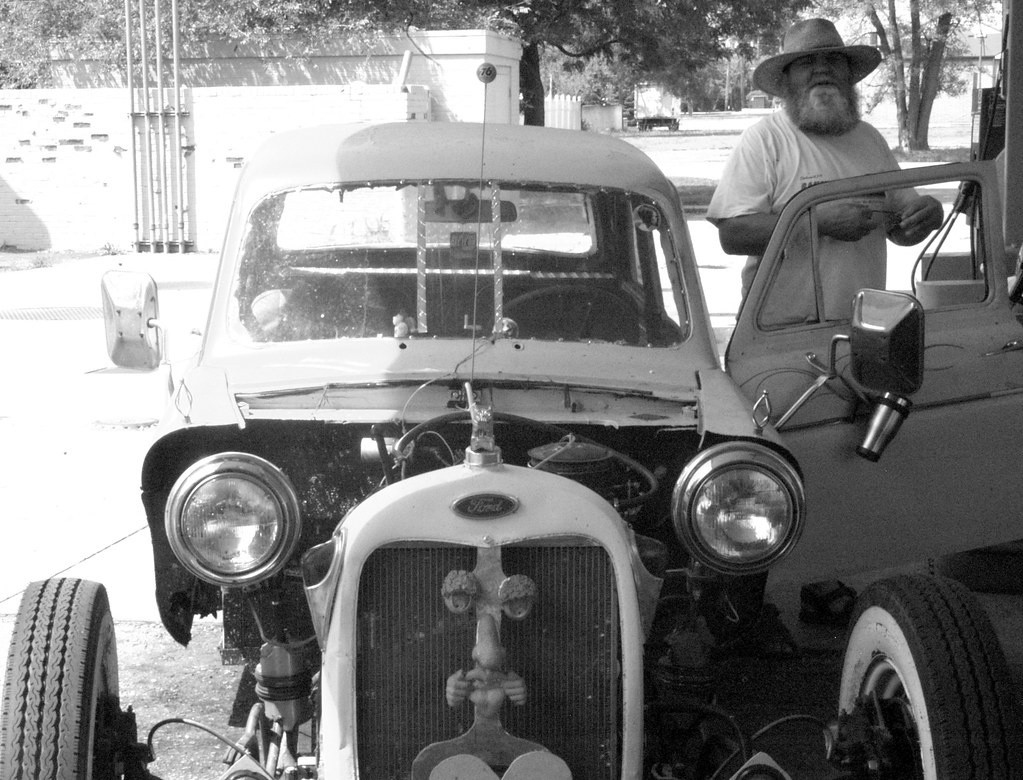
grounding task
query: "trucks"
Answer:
[634,80,682,131]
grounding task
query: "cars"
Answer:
[0,120,1023,779]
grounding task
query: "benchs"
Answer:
[290,266,634,336]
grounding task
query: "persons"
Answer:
[713,19,944,331]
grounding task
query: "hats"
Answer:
[752,18,882,97]
